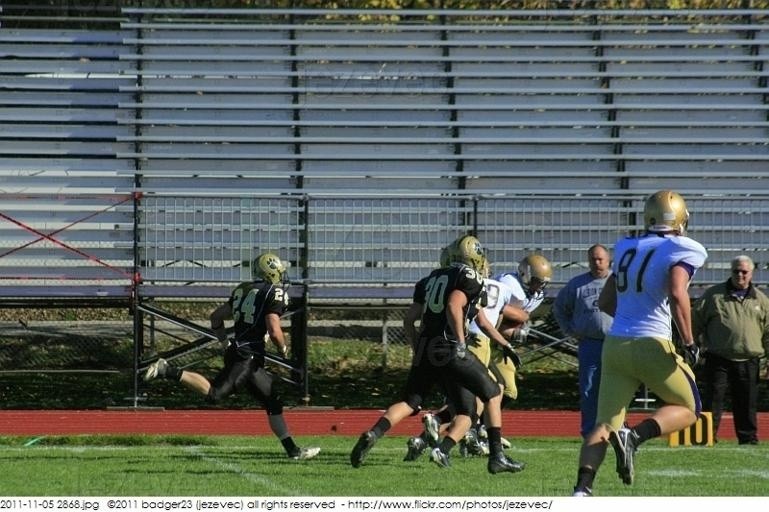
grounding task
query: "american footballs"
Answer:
[503,298,523,328]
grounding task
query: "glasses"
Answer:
[733,269,748,275]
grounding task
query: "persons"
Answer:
[691,255,769,445]
[554,244,615,439]
[352,234,553,473]
[571,190,708,496]
[143,255,321,460]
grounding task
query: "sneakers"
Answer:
[143,358,169,381]
[351,412,524,473]
[608,422,641,485]
[573,485,594,497]
[294,448,320,460]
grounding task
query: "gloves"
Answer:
[683,343,700,368]
[503,343,523,369]
[279,346,289,358]
[513,319,531,342]
[454,343,467,360]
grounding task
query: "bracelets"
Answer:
[277,346,286,353]
[210,321,223,330]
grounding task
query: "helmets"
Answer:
[440,235,489,277]
[644,191,689,237]
[254,254,291,284]
[517,255,552,300]
